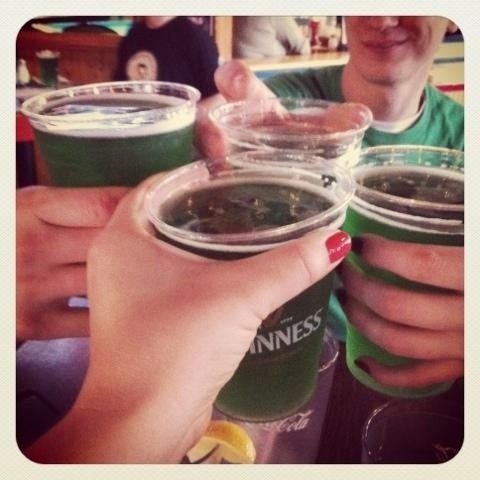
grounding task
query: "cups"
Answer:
[337,144,463,399]
[37,50,62,88]
[361,400,464,464]
[19,80,201,300]
[209,96,373,166]
[142,148,357,423]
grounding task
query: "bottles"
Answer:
[16,59,31,86]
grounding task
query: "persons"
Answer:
[15,14,465,465]
